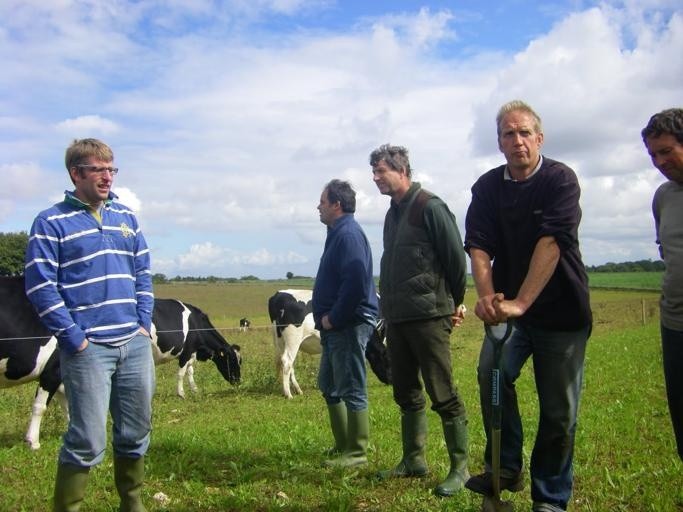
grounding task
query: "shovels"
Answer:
[484,315,514,512]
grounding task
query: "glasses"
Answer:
[77,165,119,176]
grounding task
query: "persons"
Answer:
[641,110,683,463]
[24,137,155,512]
[313,179,378,470]
[370,144,466,497]
[463,100,593,512]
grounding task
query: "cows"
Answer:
[240,319,250,332]
[151,298,242,399]
[0,276,71,450]
[268,289,387,402]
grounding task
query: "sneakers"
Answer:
[532,500,567,512]
[465,471,524,494]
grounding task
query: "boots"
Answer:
[320,401,369,469]
[114,456,148,512]
[376,415,429,477]
[435,417,471,495]
[54,464,90,512]
